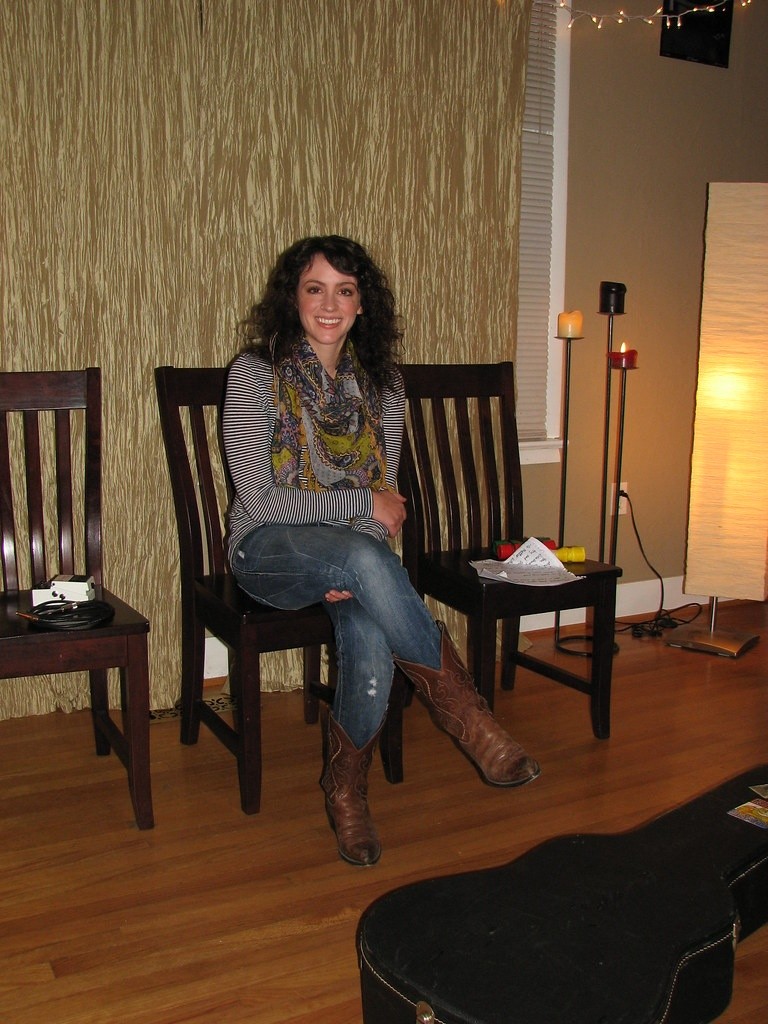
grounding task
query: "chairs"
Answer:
[150,367,407,816]
[0,365,159,831]
[390,363,625,743]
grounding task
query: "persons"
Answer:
[222,234,540,867]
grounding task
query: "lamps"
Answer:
[664,182,768,658]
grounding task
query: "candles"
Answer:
[607,343,640,371]
[596,280,627,315]
[553,311,584,340]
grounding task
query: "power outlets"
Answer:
[611,482,628,515]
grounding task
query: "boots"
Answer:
[393,620,540,787]
[321,704,388,866]
